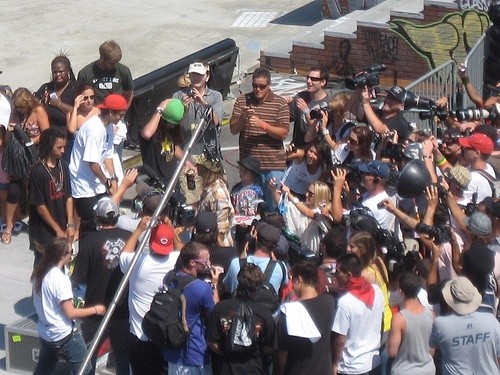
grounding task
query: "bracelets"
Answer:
[304,108,311,114]
[66,223,75,228]
[422,154,432,158]
[323,129,329,135]
[212,284,217,289]
[104,179,112,190]
[443,188,450,195]
[461,76,471,85]
[293,198,299,205]
[155,108,164,114]
[435,156,447,168]
[94,306,97,315]
[110,172,118,181]
[58,102,62,108]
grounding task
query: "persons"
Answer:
[139,62,223,204]
[269,176,500,375]
[27,126,75,297]
[329,62,500,283]
[29,168,289,375]
[229,67,333,172]
[0,40,134,243]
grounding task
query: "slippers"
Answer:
[2,225,14,244]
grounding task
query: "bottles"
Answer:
[186,169,196,190]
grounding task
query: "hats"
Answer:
[441,276,482,315]
[357,159,390,180]
[467,211,492,236]
[188,62,212,76]
[93,197,119,218]
[385,85,407,102]
[236,157,262,177]
[256,222,281,244]
[97,94,128,110]
[160,98,185,125]
[196,210,218,233]
[443,164,473,192]
[149,224,175,255]
[191,153,222,173]
[458,133,494,155]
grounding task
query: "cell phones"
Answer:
[50,93,58,99]
[378,201,384,210]
[245,96,252,110]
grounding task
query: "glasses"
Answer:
[194,259,211,266]
[83,94,95,100]
[464,147,477,152]
[307,75,325,82]
[252,83,269,90]
[346,136,360,146]
[305,188,315,197]
[64,248,74,255]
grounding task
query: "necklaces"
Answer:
[44,159,62,192]
[39,159,61,184]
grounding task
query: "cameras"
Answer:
[403,92,495,123]
[330,163,360,181]
[464,193,478,215]
[205,264,214,274]
[272,180,283,190]
[185,86,193,96]
[418,223,453,245]
[344,65,387,89]
[309,107,333,120]
[449,130,471,142]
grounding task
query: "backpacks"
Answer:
[474,163,500,219]
[142,271,197,350]
[227,303,260,358]
[240,258,278,313]
